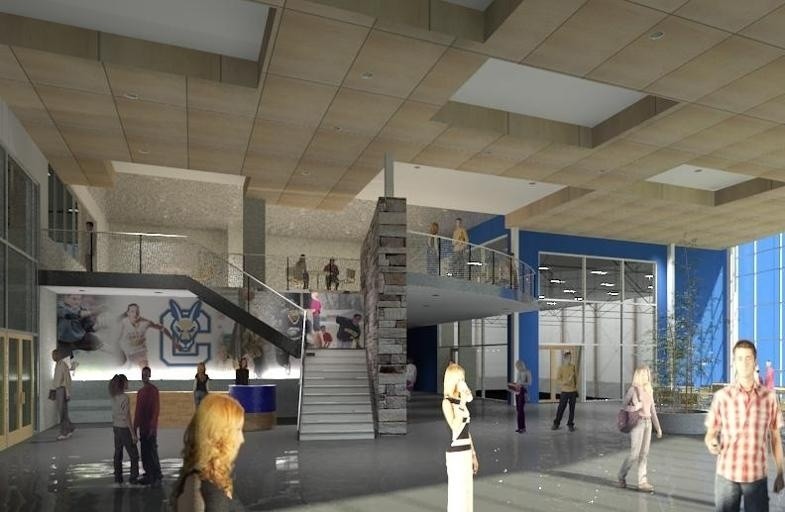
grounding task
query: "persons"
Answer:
[105,371,140,488]
[133,366,164,481]
[295,216,474,389]
[617,362,662,493]
[550,351,578,432]
[764,360,775,391]
[169,393,254,512]
[192,361,210,410]
[704,339,785,512]
[507,359,532,432]
[236,355,252,388]
[440,364,479,512]
[47,347,79,440]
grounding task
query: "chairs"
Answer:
[287,268,357,292]
[651,381,785,427]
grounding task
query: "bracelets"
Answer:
[458,407,464,411]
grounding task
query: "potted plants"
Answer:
[217,330,266,384]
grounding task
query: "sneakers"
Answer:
[71,427,79,434]
[639,482,654,489]
[551,424,559,430]
[569,425,575,432]
[619,479,627,488]
[151,478,162,488]
[128,482,148,489]
[113,482,128,489]
[56,432,72,441]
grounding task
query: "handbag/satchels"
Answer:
[617,401,638,433]
